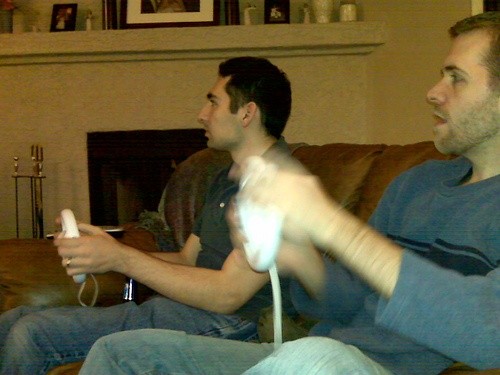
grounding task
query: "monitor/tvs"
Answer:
[86,128,209,227]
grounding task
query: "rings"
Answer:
[67,258,71,267]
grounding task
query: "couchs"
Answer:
[0,142,500,375]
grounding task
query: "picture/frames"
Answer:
[50,3,78,32]
[119,0,221,29]
[263,0,290,25]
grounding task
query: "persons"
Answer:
[0,56,313,373]
[77,11,500,375]
